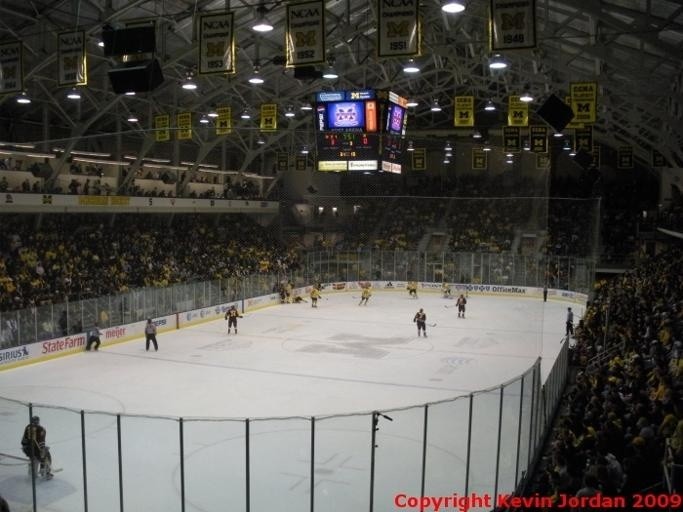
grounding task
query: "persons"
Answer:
[0,142,681,352]
[518,352,683,511]
[19,414,54,481]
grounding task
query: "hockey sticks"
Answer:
[444,304,457,309]
[425,323,436,327]
[35,441,63,472]
